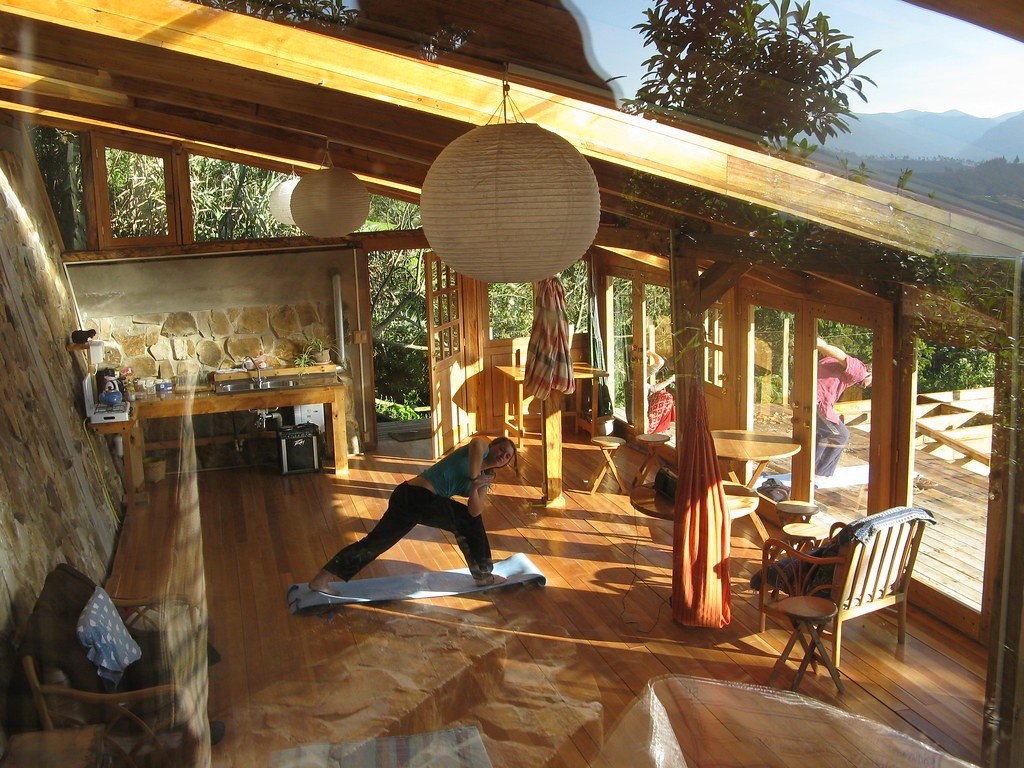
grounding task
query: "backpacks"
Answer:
[757,476,793,503]
[587,382,614,417]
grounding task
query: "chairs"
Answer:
[22,564,214,768]
[511,338,541,438]
[759,506,936,667]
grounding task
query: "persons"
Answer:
[646,351,676,434]
[815,337,872,487]
[308,436,517,596]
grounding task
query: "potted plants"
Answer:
[296,326,351,377]
[591,376,615,436]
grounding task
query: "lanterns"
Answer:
[291,169,369,238]
[420,123,600,282]
[270,180,297,226]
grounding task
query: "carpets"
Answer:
[266,726,494,768]
[388,428,432,442]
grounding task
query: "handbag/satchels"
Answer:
[653,466,678,503]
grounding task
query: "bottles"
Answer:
[258,352,266,369]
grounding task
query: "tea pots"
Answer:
[99,380,123,404]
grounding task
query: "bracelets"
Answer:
[469,476,479,481]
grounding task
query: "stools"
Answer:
[769,596,846,694]
[586,436,627,494]
[631,434,670,487]
[775,500,829,549]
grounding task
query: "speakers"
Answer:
[281,428,319,475]
[654,466,678,502]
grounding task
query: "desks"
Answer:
[496,363,609,447]
[0,724,105,768]
[630,479,759,520]
[710,429,801,545]
[87,376,356,516]
